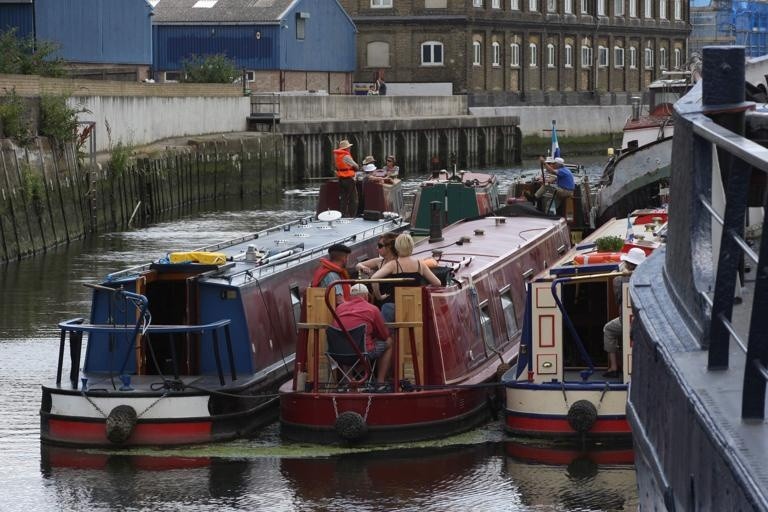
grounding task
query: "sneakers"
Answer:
[602,370,619,378]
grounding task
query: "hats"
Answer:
[328,244,351,253]
[337,140,353,150]
[364,164,377,171]
[620,248,646,265]
[350,284,373,300]
[363,156,377,164]
[544,157,565,164]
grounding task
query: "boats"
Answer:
[500,208,670,440]
[37,440,252,512]
[492,431,645,510]
[34,205,411,451]
[275,440,498,512]
[275,198,570,446]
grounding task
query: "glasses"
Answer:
[377,243,385,248]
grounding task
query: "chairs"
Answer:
[325,323,377,392]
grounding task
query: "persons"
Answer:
[368,78,386,95]
[311,244,352,303]
[354,233,398,279]
[334,138,399,217]
[369,233,441,376]
[602,247,647,378]
[331,283,393,389]
[523,156,575,210]
